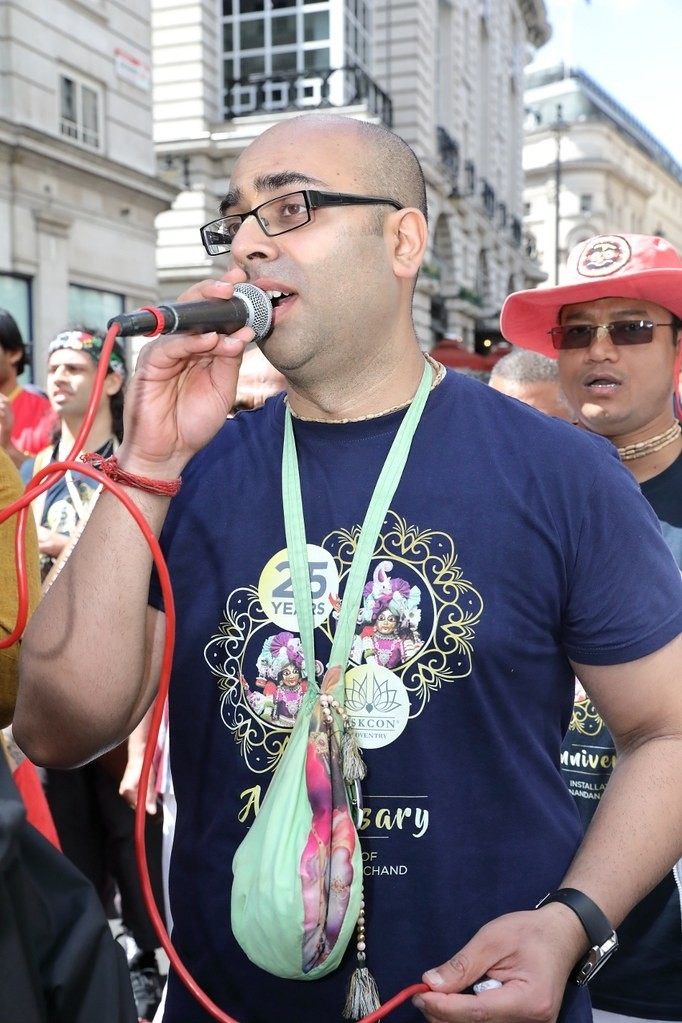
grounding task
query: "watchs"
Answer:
[541,889,618,986]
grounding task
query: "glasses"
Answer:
[547,319,680,350]
[198,189,406,257]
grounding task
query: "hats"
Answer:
[499,234,682,376]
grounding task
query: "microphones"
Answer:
[105,283,274,343]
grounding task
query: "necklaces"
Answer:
[616,418,679,452]
[60,438,112,484]
[283,350,446,425]
[618,424,679,456]
[621,425,682,460]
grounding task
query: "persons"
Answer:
[0,112,682,1023]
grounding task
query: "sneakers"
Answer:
[129,958,162,1021]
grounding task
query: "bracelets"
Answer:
[80,452,182,497]
